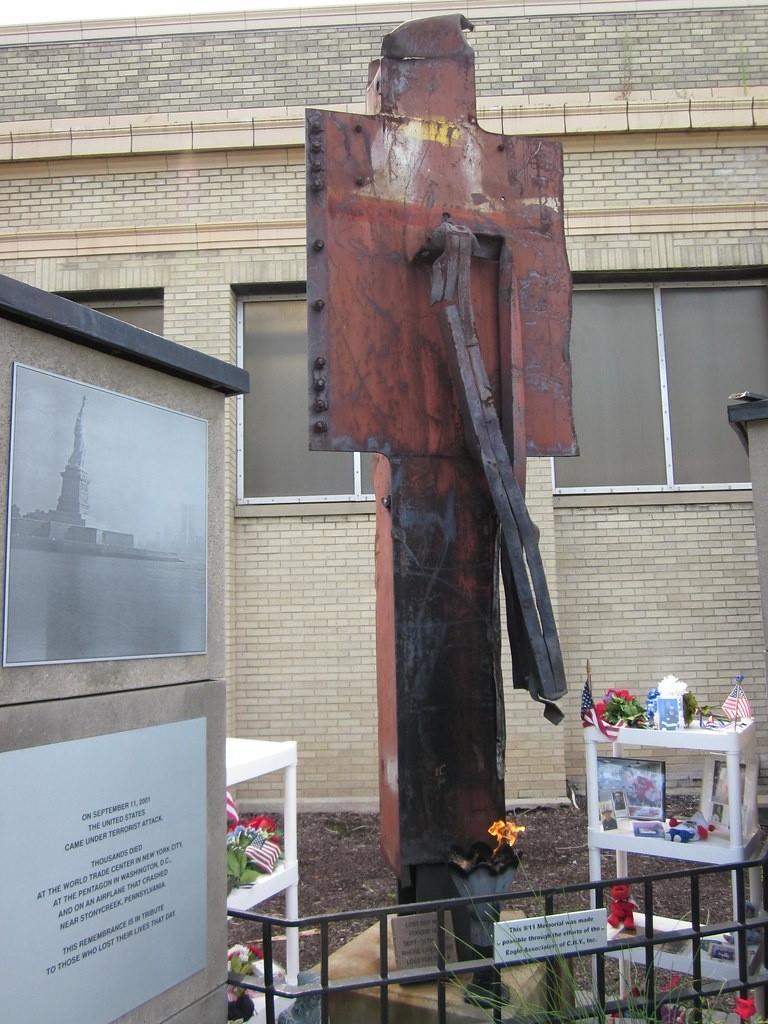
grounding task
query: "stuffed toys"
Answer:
[608,882,637,932]
[666,811,716,842]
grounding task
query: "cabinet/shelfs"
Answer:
[229,733,307,1024]
[581,702,767,990]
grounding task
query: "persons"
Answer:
[67,395,88,469]
[614,793,625,809]
[616,766,655,807]
[601,810,617,830]
[711,806,721,823]
